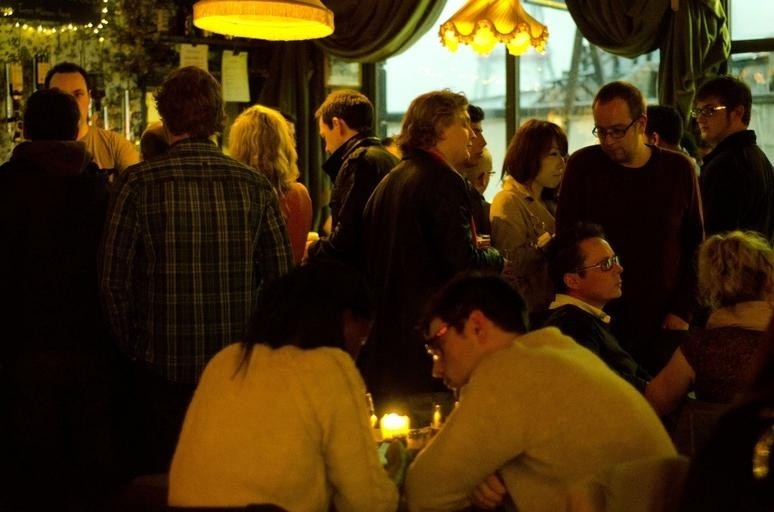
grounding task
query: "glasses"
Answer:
[691,106,726,118]
[424,323,451,362]
[592,114,643,139]
[576,255,619,272]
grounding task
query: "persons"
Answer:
[0,62,771,512]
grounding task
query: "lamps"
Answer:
[437,0,549,56]
[192,0,336,42]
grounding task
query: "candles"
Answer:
[379,412,410,440]
